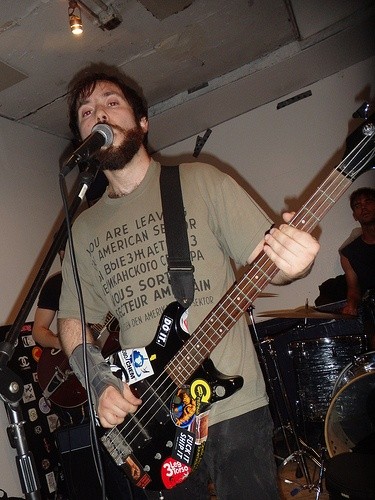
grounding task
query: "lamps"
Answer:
[70,16,83,34]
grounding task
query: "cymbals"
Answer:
[255,293,280,298]
[256,306,360,319]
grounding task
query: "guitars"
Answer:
[86,96,375,492]
[37,307,122,409]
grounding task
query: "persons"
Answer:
[56,74,320,500]
[340,188,375,353]
[31,224,124,351]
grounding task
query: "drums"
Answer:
[294,331,375,458]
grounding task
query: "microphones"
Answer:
[66,122,116,174]
